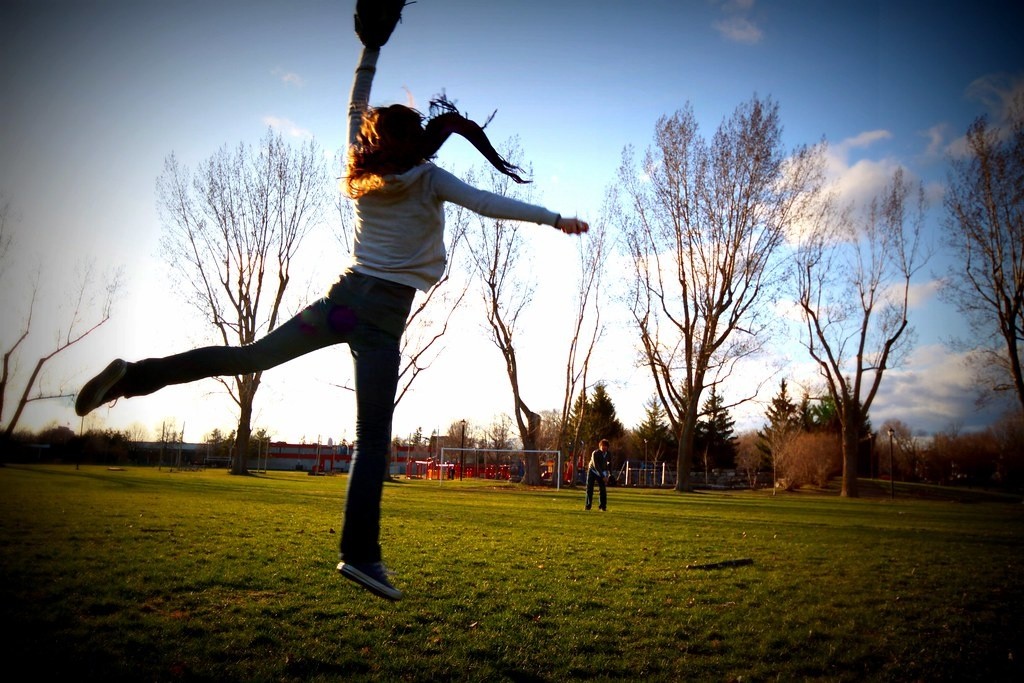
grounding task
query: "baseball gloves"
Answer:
[354,0,418,49]
[607,474,617,487]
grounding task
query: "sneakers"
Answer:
[337,553,402,601]
[75,359,131,416]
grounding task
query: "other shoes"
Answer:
[599,506,606,511]
[585,506,591,510]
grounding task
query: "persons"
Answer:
[586,439,610,510]
[74,44,589,602]
